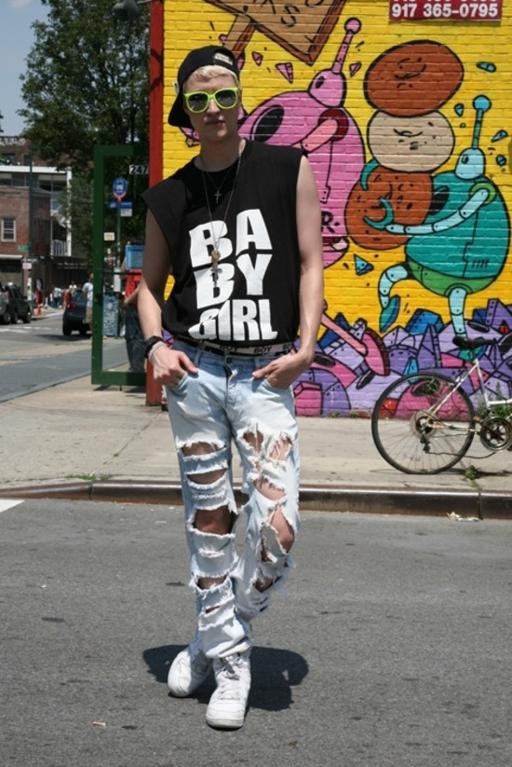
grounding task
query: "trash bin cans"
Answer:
[102,292,124,336]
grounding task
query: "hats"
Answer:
[167,45,239,131]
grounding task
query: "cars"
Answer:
[62,290,89,337]
[0,282,33,325]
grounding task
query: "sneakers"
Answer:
[167,637,214,699]
[205,656,252,729]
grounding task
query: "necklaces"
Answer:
[190,140,244,281]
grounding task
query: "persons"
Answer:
[17,271,113,319]
[137,47,325,731]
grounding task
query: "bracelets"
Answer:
[141,334,166,360]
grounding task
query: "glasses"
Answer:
[178,86,239,115]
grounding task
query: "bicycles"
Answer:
[371,335,512,476]
